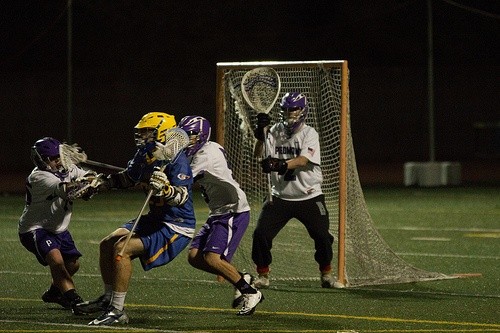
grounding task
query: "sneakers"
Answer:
[237,291,265,315]
[88,304,129,326]
[321,275,345,289]
[232,274,255,308]
[58,289,84,309]
[72,294,110,315]
[252,276,270,290]
[42,290,60,303]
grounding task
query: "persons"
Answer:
[17,136,98,316]
[70,112,196,327]
[251,91,346,290]
[178,114,265,317]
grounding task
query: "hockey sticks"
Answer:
[59,143,126,171]
[116,128,189,261]
[241,68,281,201]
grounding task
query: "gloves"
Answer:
[150,166,170,197]
[260,157,288,175]
[68,174,103,200]
[253,113,272,141]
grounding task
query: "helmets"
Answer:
[278,92,306,130]
[31,136,62,172]
[178,116,212,157]
[134,112,177,164]
